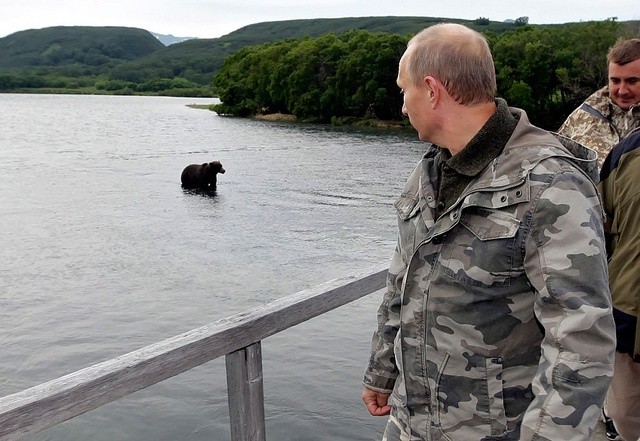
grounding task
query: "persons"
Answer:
[600,129,639,439]
[556,37,640,179]
[362,22,618,440]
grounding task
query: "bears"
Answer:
[181,161,226,192]
